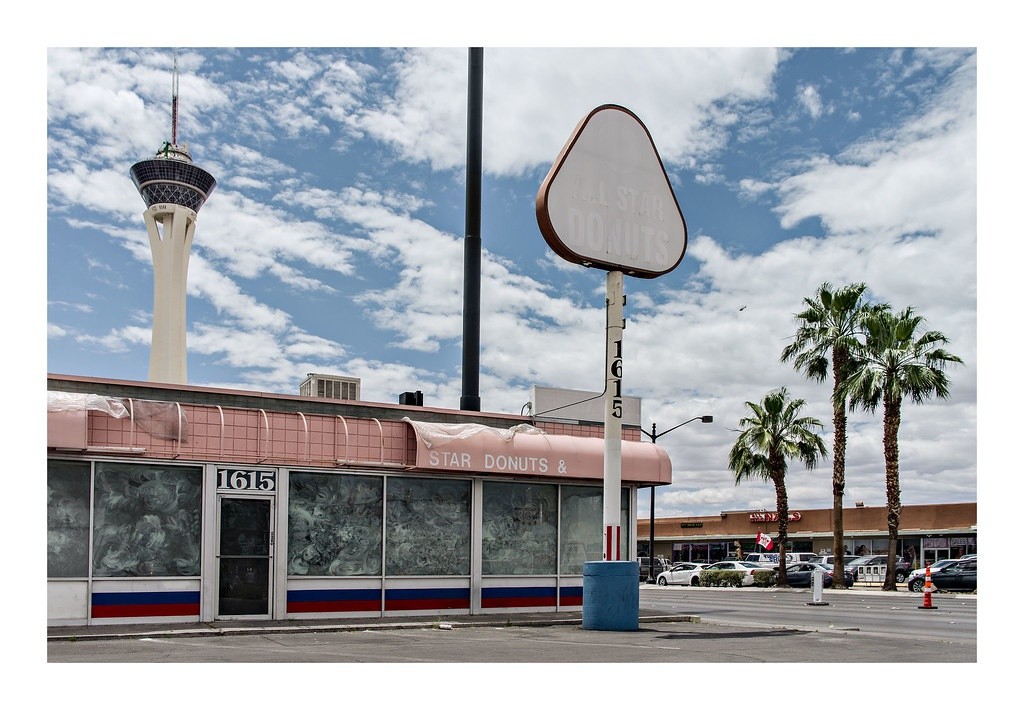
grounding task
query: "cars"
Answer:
[655,562,713,587]
[908,553,976,592]
[704,561,776,587]
[847,555,911,583]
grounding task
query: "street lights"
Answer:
[640,415,714,581]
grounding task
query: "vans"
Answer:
[744,552,820,579]
[636,556,673,582]
[805,555,861,570]
[773,560,854,588]
